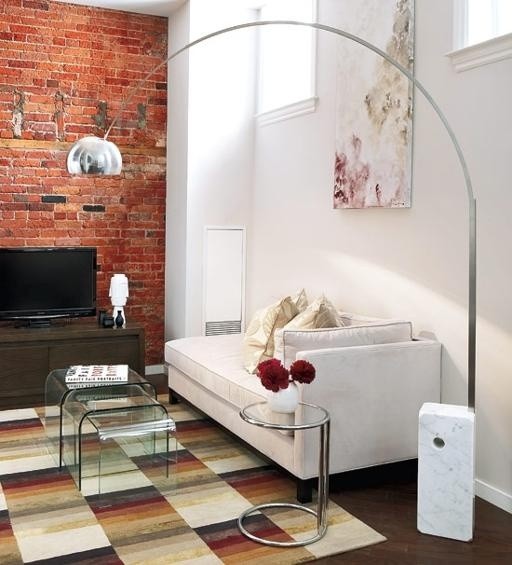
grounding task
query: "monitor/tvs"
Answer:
[0,246,97,330]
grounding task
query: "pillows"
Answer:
[242,287,413,376]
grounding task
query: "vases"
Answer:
[265,382,300,414]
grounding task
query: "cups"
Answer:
[99,310,106,325]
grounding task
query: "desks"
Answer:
[43,364,179,511]
[236,402,333,549]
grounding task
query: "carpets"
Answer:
[1,391,388,565]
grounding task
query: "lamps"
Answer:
[64,17,485,544]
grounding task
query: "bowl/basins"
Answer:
[103,317,114,327]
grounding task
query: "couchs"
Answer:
[163,309,444,509]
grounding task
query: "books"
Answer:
[65,382,127,389]
[65,364,129,382]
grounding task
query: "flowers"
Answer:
[257,358,316,394]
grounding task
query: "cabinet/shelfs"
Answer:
[1,318,146,413]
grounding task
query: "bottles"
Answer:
[115,310,123,327]
